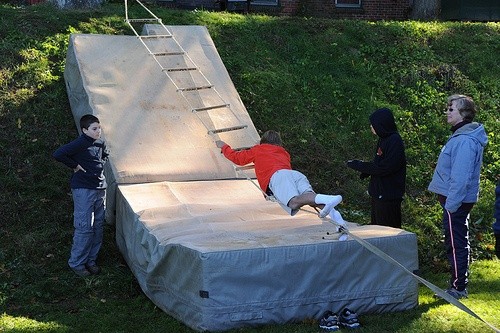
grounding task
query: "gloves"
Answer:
[345,159,364,170]
[357,171,369,179]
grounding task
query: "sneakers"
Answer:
[337,308,360,328]
[319,310,340,330]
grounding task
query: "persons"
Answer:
[216,130,348,241]
[428,95,489,298]
[348,107,406,228]
[55,114,109,276]
[492,184,500,259]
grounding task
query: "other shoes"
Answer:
[70,264,101,278]
[433,286,469,301]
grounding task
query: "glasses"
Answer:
[445,108,458,112]
